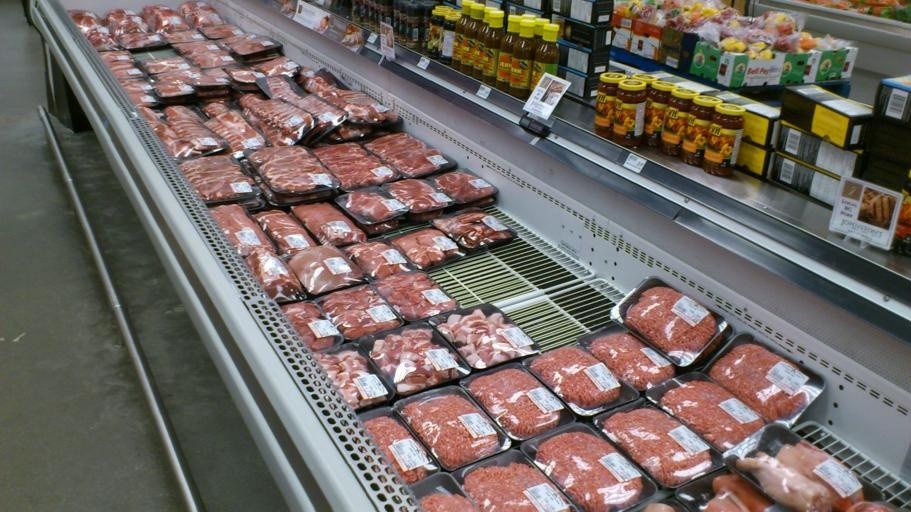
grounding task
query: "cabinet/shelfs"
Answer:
[29,0,911,512]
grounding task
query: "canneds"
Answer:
[594,72,746,177]
[427,5,461,65]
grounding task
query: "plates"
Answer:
[317,276,907,512]
[87,24,283,105]
[141,68,517,354]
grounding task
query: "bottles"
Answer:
[329,0,462,65]
[592,72,745,180]
[450,0,560,101]
